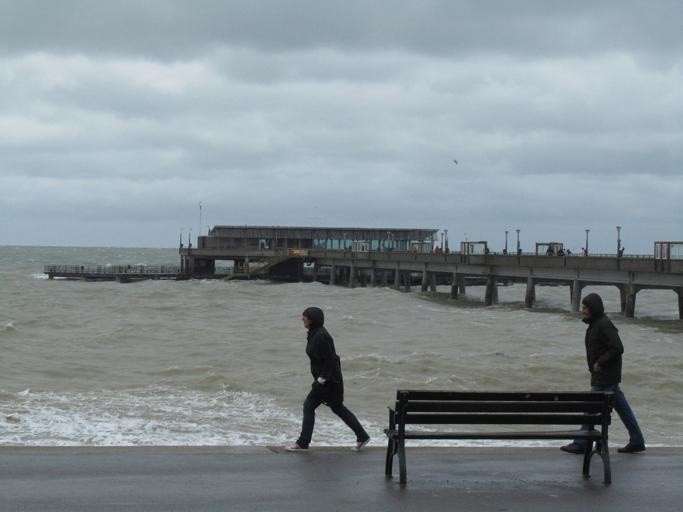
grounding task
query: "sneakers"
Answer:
[351,437,371,451]
[285,442,309,452]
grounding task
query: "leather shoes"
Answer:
[560,442,587,454]
[618,443,645,453]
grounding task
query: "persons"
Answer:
[546,246,555,256]
[559,292,645,453]
[283,307,372,453]
[581,248,585,257]
[567,249,572,256]
[413,245,418,253]
[558,249,565,257]
[446,247,449,253]
[435,246,441,252]
[620,247,625,256]
[483,245,489,254]
[583,250,588,256]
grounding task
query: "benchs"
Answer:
[382,387,617,487]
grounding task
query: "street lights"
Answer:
[441,228,450,252]
[582,230,590,255]
[503,230,508,254]
[516,229,522,254]
[617,226,625,257]
